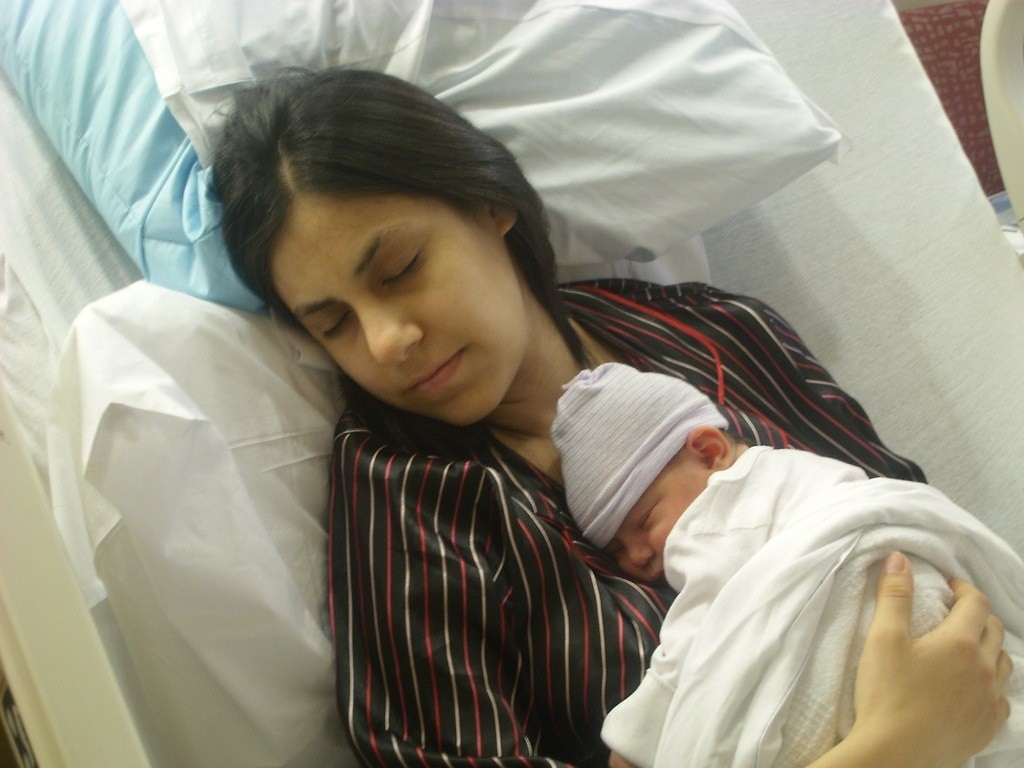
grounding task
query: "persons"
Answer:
[549,361,1024,768]
[207,64,929,768]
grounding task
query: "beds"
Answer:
[0,0,1024,768]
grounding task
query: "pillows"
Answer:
[0,0,839,309]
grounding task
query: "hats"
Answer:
[549,363,729,549]
